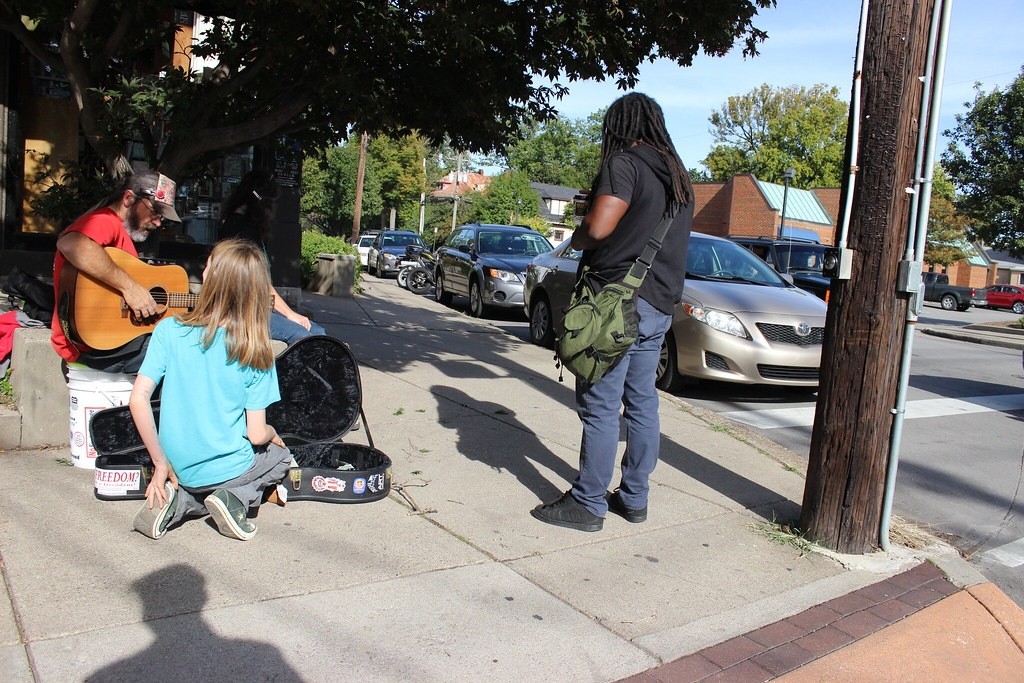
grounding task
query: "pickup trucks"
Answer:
[921,271,988,311]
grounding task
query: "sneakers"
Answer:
[205,489,258,540]
[132,479,179,540]
[531,491,603,532]
[604,487,647,522]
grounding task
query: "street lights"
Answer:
[780,166,795,238]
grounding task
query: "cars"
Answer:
[523,233,835,394]
[974,284,1024,313]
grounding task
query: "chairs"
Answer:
[511,239,527,254]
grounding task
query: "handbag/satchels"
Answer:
[555,283,641,384]
[0,265,55,328]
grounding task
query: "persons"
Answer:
[52,170,193,376]
[129,237,291,540]
[217,169,325,345]
[533,92,694,531]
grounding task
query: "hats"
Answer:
[126,171,182,224]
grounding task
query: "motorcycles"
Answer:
[396,234,450,295]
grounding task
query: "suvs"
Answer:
[352,227,432,278]
[434,220,555,318]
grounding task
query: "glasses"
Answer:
[140,198,164,221]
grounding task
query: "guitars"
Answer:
[56,244,276,354]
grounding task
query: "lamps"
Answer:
[38,38,59,48]
